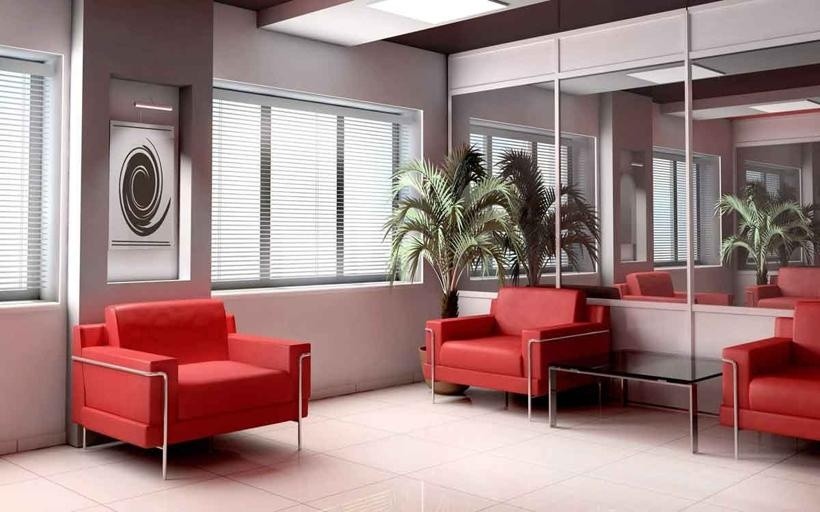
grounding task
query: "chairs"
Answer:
[612,272,731,304]
[743,266,820,309]
[72,299,311,479]
[721,302,819,443]
[426,288,611,418]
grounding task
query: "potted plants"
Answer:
[381,142,526,394]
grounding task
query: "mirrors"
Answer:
[560,59,687,301]
[691,38,820,306]
[447,77,556,282]
